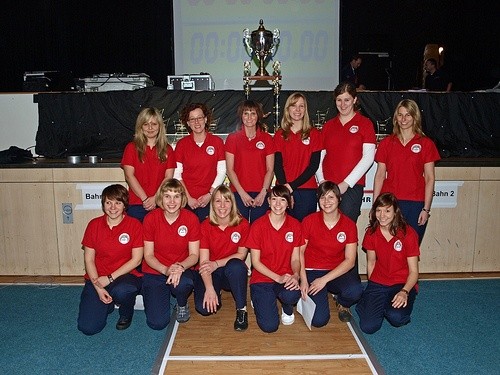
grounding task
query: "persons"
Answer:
[195,185,251,331]
[246,186,305,333]
[314,82,377,223]
[372,99,440,243]
[425,58,452,91]
[142,179,200,331]
[273,93,320,219]
[175,105,227,222]
[299,181,362,327]
[122,106,177,220]
[225,105,276,224]
[358,192,420,334]
[78,184,144,335]
[342,55,366,90]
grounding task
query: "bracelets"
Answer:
[177,263,185,269]
[423,209,430,214]
[400,289,409,296]
[143,197,149,203]
[108,274,113,283]
[209,191,212,196]
[263,188,268,192]
[214,260,220,268]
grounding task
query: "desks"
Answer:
[35,91,500,159]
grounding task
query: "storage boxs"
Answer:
[79,73,154,92]
[167,75,215,91]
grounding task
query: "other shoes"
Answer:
[281,307,295,325]
[107,302,115,315]
[336,301,352,322]
[116,315,133,330]
[176,303,190,322]
[234,310,249,331]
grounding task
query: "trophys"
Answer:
[244,19,281,91]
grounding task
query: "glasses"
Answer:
[187,115,206,122]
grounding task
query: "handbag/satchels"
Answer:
[0,146,33,164]
